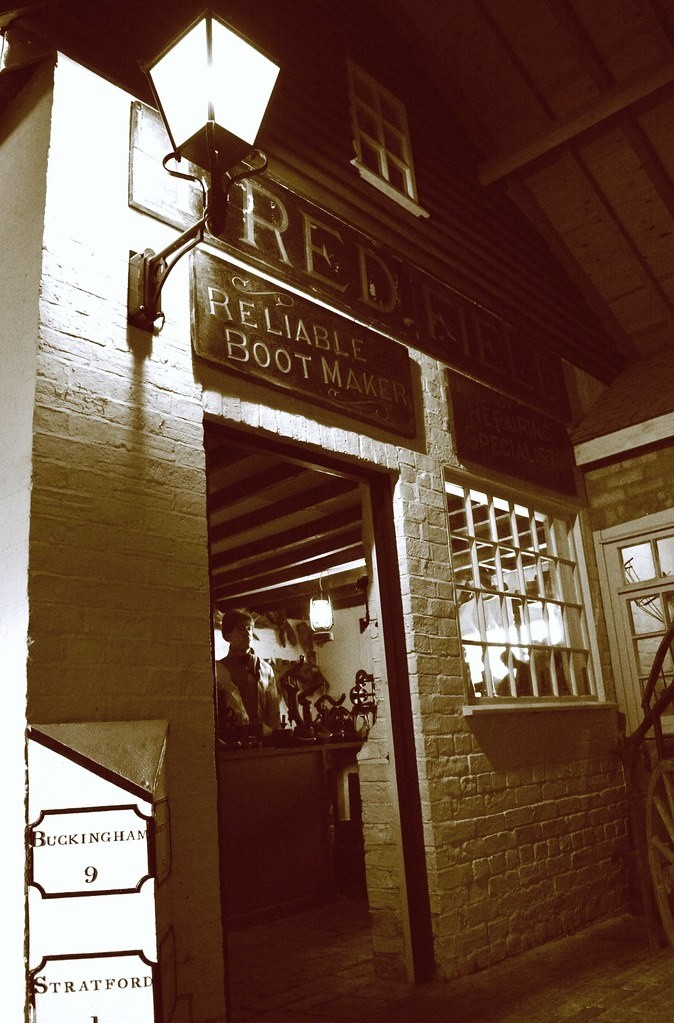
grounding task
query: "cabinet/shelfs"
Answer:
[220,738,359,932]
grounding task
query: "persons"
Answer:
[214,603,284,742]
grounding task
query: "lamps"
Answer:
[122,5,288,328]
[308,571,336,641]
